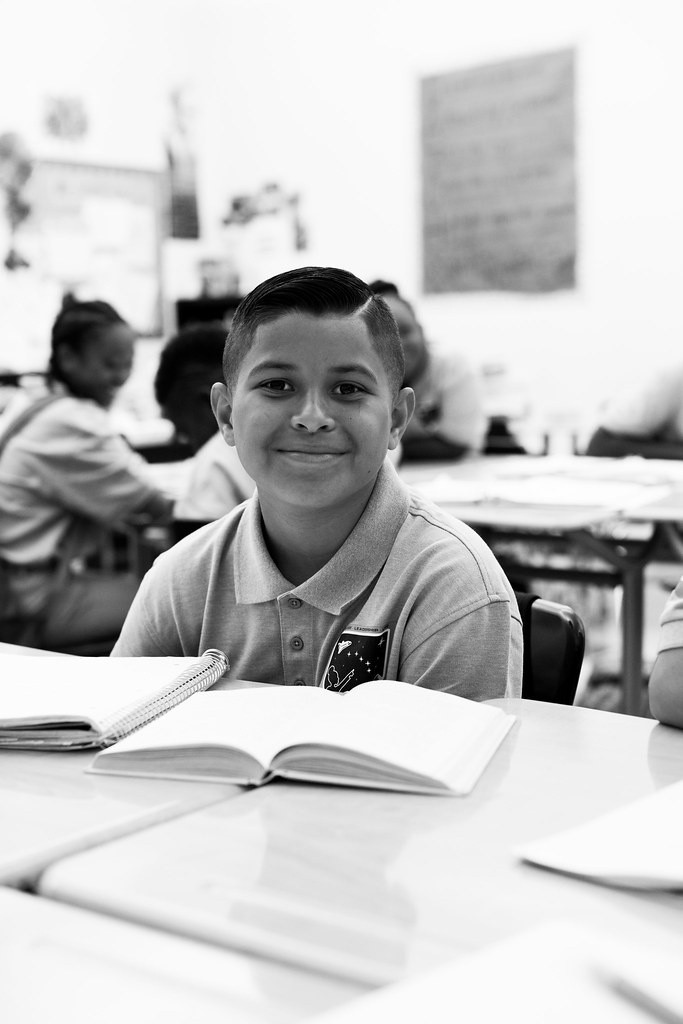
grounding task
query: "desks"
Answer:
[39,695,683,1024]
[0,640,276,888]
[0,885,376,1024]
[396,456,683,718]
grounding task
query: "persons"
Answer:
[109,268,525,703]
[366,280,492,465]
[155,320,257,545]
[648,577,683,729]
[0,294,174,649]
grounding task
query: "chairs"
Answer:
[512,594,588,706]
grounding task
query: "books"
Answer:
[0,641,231,752]
[82,679,517,798]
[518,778,682,891]
